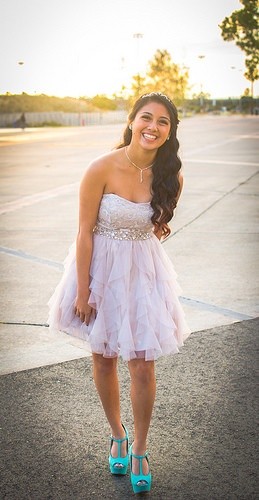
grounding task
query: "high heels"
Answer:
[108,423,130,474]
[129,440,152,492]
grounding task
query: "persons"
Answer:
[46,94,192,494]
[21,113,25,129]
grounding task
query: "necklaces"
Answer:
[125,146,156,183]
